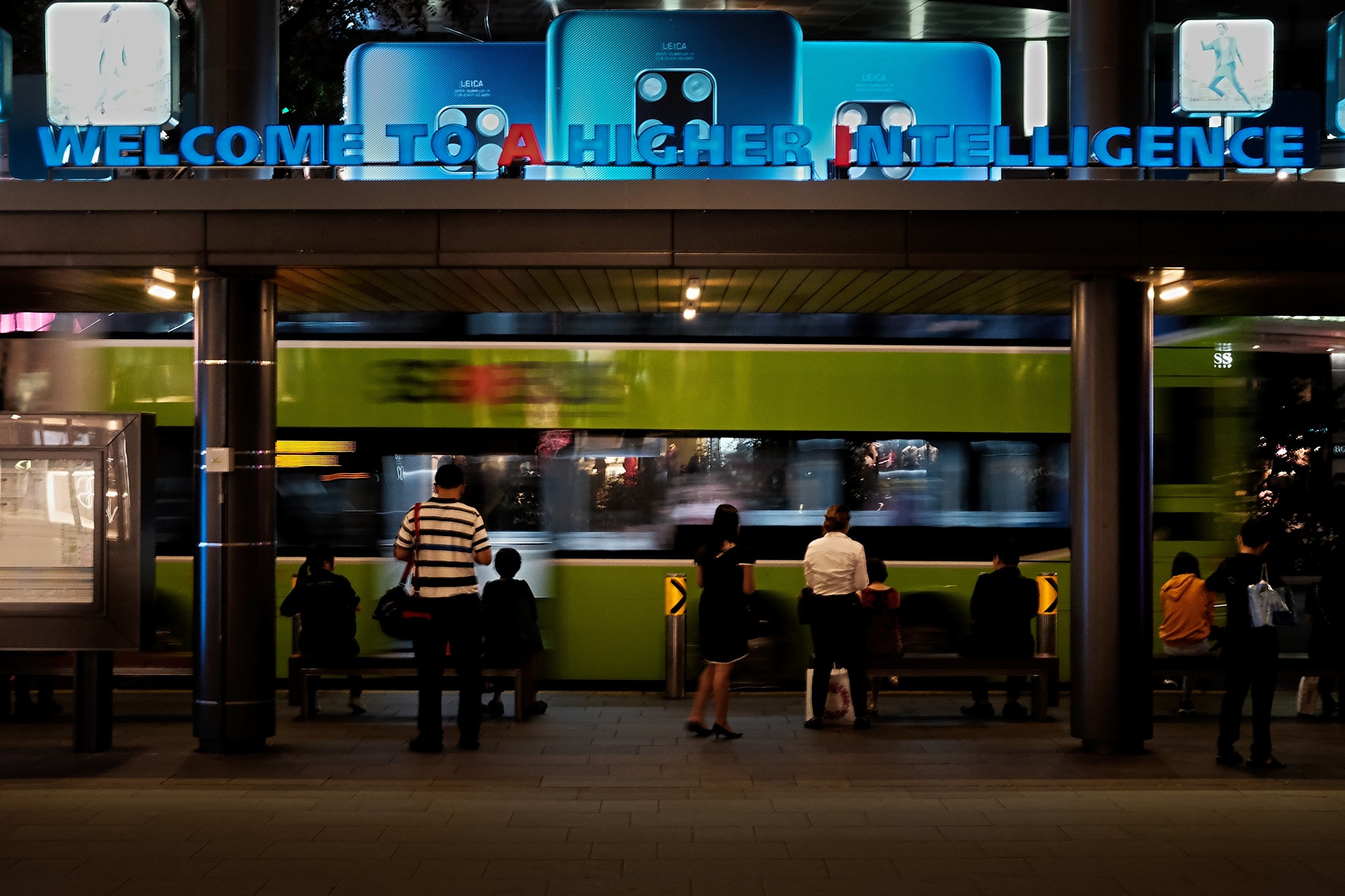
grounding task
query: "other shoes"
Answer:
[1003,701,1028,717]
[1216,751,1244,767]
[488,700,504,719]
[960,701,995,718]
[409,739,443,753]
[1246,755,1283,771]
[522,701,548,714]
[457,739,480,751]
[854,718,871,730]
[804,719,823,729]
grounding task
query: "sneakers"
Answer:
[348,696,366,712]
[1164,677,1179,687]
[1178,698,1196,713]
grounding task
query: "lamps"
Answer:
[683,278,701,321]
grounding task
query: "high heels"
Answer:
[687,721,712,736]
[712,723,743,739]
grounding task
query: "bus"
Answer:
[0,316,1345,684]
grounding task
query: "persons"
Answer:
[481,546,547,716]
[1305,554,1341,719]
[803,506,872,733]
[1160,550,1216,715]
[683,506,758,741]
[960,541,1040,722]
[434,429,937,523]
[279,543,367,721]
[856,556,901,718]
[1203,519,1294,771]
[394,465,492,754]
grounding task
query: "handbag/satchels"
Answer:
[797,587,817,625]
[1248,583,1300,633]
[805,662,856,727]
[370,585,430,640]
[1297,675,1320,715]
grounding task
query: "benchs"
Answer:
[298,661,525,724]
[868,652,1061,725]
[0,650,196,723]
[1154,651,1344,723]
